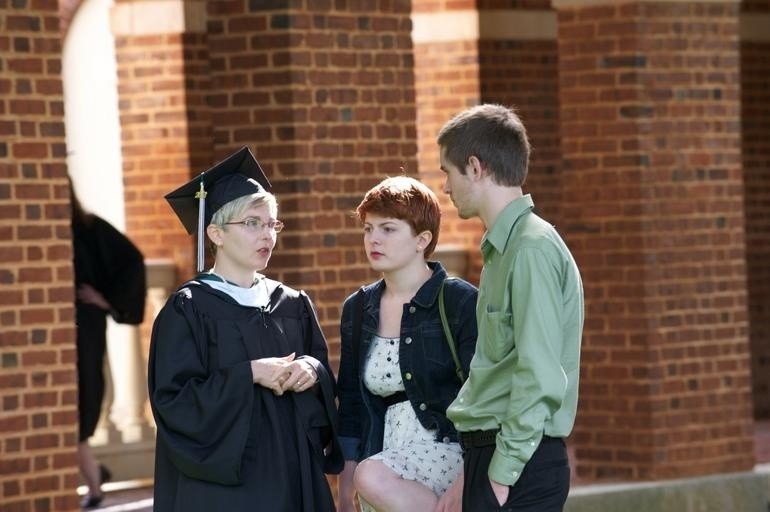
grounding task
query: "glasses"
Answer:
[222,218,283,234]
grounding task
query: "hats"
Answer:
[164,145,271,271]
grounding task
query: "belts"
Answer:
[462,429,499,448]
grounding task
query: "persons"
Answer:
[147,146,345,512]
[334,174,480,511]
[436,104,586,511]
[65,174,148,512]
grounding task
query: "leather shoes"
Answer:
[82,463,111,508]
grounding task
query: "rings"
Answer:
[297,380,303,385]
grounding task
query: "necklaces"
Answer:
[209,268,228,284]
